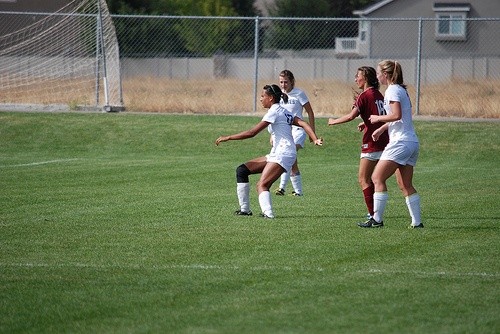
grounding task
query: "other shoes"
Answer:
[257,212,274,220]
[291,190,302,197]
[234,209,253,216]
[275,188,286,196]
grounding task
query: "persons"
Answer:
[328,66,389,226]
[215,84,324,219]
[268,70,318,196]
[357,59,424,230]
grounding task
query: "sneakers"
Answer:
[356,217,384,228]
[407,222,424,229]
